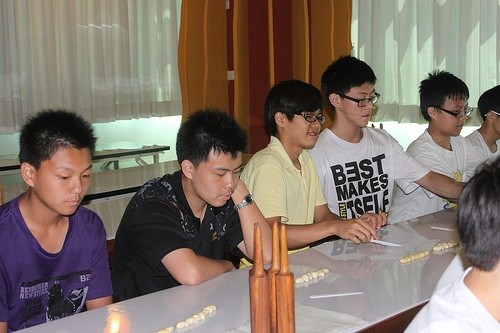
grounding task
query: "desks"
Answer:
[0,153,255,241]
[11,203,463,333]
[0,145,170,177]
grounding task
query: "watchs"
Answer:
[234,194,254,210]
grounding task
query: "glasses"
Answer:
[336,92,380,107]
[293,111,325,125]
[431,105,474,119]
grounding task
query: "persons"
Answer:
[239,81,387,268]
[419,244,460,298]
[304,55,470,248]
[0,109,114,333]
[464,85,500,159]
[291,250,375,278]
[403,156,500,333]
[388,71,485,225]
[399,207,462,242]
[112,107,273,303]
